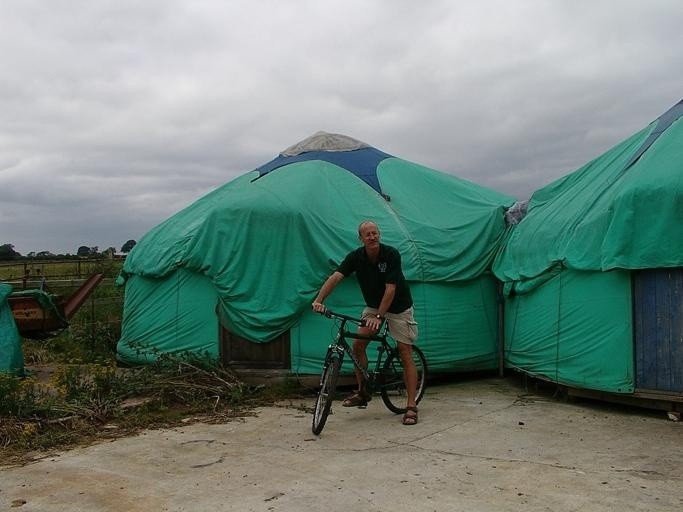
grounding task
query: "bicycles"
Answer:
[308,304,429,435]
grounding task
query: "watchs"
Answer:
[374,313,384,321]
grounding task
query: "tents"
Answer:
[491,98,680,420]
[115,130,516,390]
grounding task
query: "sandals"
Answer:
[342,392,368,407]
[402,405,419,425]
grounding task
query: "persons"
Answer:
[311,219,418,425]
